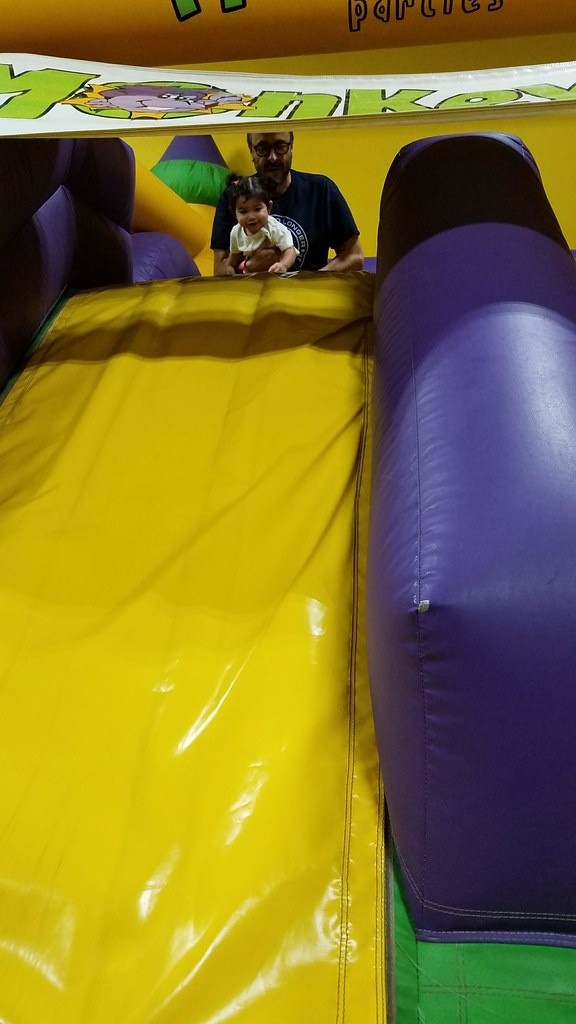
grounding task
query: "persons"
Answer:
[210,132,364,277]
[227,172,300,274]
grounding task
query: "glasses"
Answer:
[251,141,292,157]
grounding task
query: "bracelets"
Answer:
[240,260,251,275]
